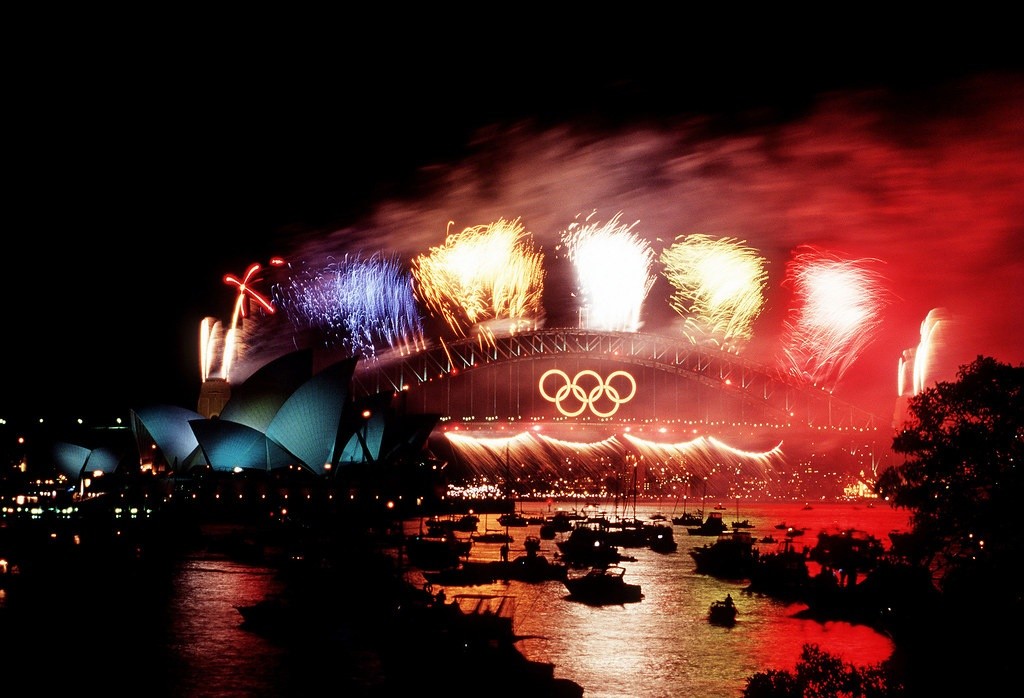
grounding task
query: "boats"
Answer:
[403,439,886,626]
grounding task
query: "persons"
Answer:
[725,594,734,608]
[725,598,734,608]
